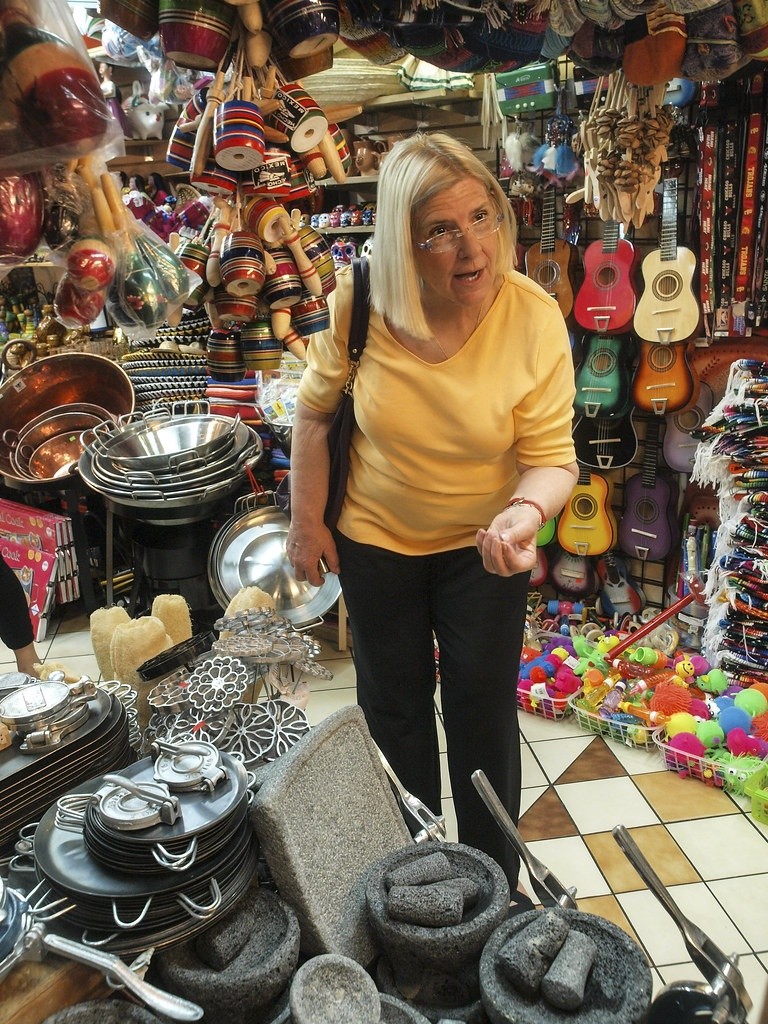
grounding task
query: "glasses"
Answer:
[411,193,504,254]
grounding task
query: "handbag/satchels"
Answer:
[275,256,372,532]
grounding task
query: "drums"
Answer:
[1,0,350,382]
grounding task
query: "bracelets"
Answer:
[506,498,547,532]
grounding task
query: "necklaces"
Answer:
[428,303,484,360]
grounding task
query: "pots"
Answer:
[0,353,342,631]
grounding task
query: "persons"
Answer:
[0,554,44,680]
[287,129,576,902]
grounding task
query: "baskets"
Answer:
[511,632,583,721]
[743,760,768,827]
[651,725,768,789]
[565,685,663,751]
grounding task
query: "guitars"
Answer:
[527,464,647,620]
[572,334,635,418]
[573,218,641,335]
[633,156,700,346]
[618,415,680,563]
[629,340,701,416]
[571,380,639,469]
[525,183,578,322]
[661,381,715,475]
